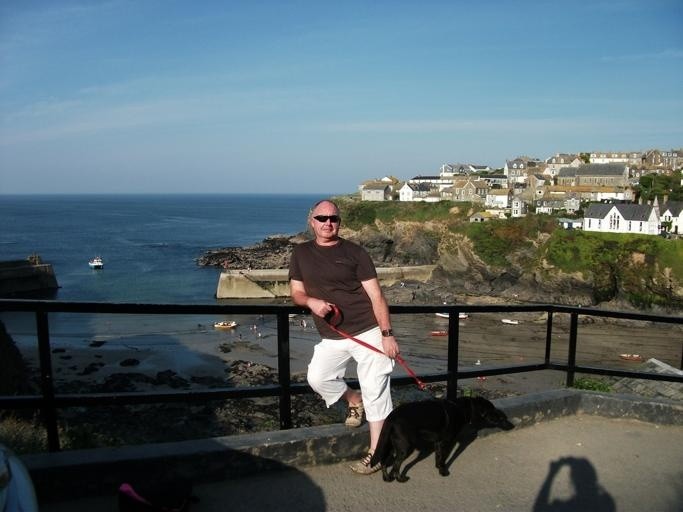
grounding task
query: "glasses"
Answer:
[313,215,341,226]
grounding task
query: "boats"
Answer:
[619,354,641,361]
[0,251,61,299]
[214,323,238,328]
[501,319,518,325]
[88,257,103,269]
[431,328,448,336]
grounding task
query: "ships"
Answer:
[435,312,468,319]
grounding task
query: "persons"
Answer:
[116,435,200,512]
[288,199,400,475]
[0,438,39,512]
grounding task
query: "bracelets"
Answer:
[381,329,394,337]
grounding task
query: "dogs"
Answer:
[370,395,516,483]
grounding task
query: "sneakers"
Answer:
[348,448,382,475]
[345,389,364,428]
